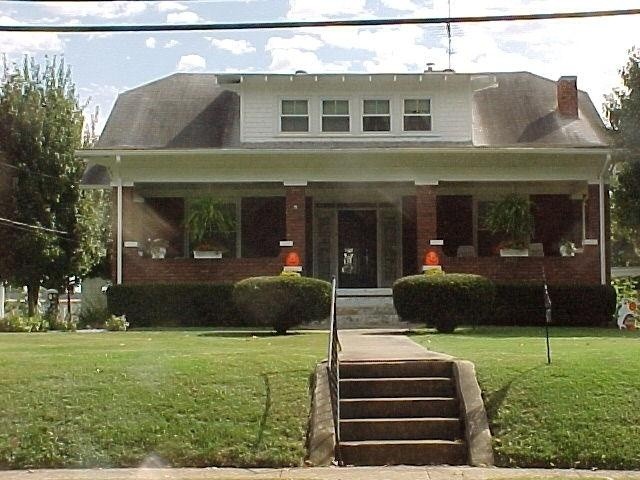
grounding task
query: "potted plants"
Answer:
[485,192,537,257]
[558,237,576,256]
[182,192,239,259]
[144,229,171,260]
